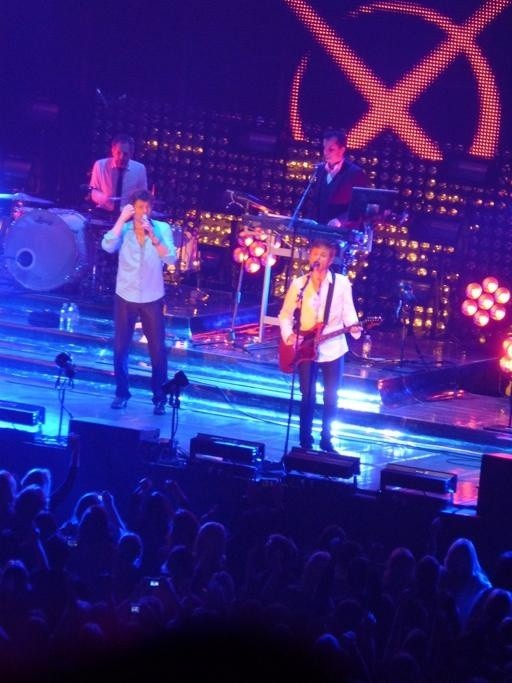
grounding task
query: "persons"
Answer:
[2,436,509,683]
[100,189,178,415]
[88,133,150,216]
[296,127,372,232]
[280,238,363,452]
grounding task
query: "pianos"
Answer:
[246,213,368,248]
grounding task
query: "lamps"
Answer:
[449,263,512,377]
[231,228,283,276]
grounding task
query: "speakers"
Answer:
[475,452,512,518]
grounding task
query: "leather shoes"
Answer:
[111,395,128,409]
[153,403,165,415]
[320,442,341,456]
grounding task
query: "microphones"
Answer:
[312,162,327,170]
[309,262,319,271]
[141,212,150,234]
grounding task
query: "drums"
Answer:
[5,207,89,293]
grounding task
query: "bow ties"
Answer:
[327,162,337,170]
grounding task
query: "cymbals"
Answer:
[151,209,177,219]
[0,193,53,204]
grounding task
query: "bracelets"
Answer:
[151,238,161,246]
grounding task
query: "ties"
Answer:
[113,168,122,216]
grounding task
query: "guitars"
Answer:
[279,317,383,372]
